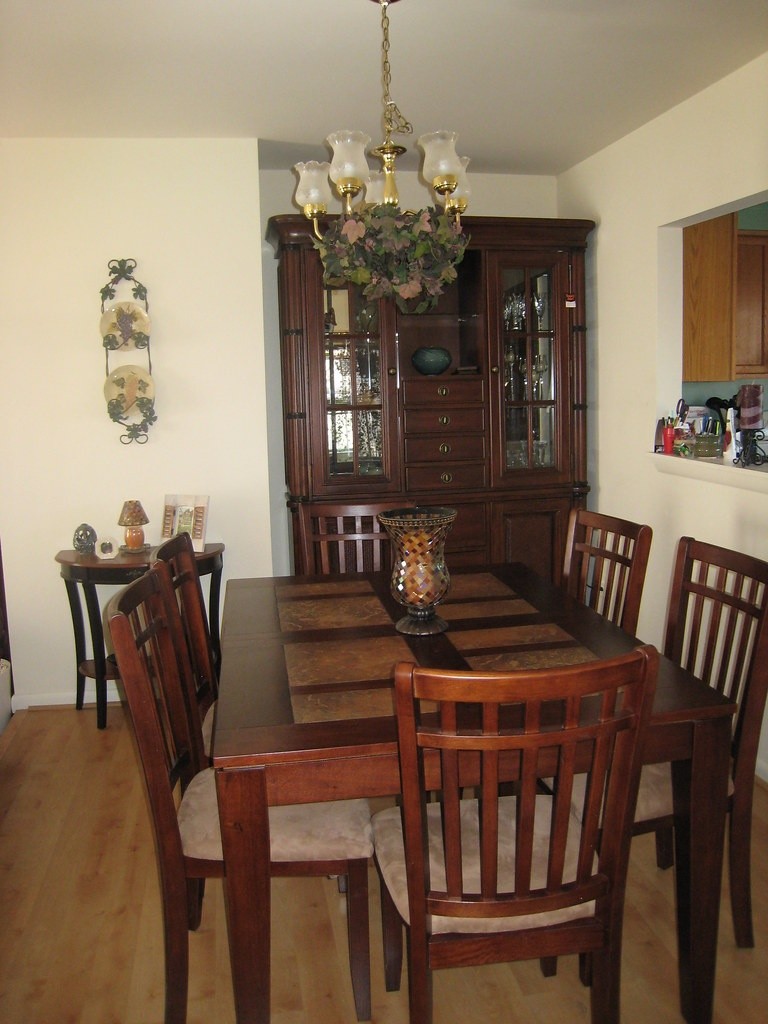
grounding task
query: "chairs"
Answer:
[102,573,375,1023]
[284,500,422,577]
[375,643,654,1024]
[565,506,655,637]
[155,532,346,888]
[536,537,768,1024]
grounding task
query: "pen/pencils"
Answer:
[661,417,682,428]
[699,417,720,436]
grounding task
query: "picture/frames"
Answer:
[160,495,208,553]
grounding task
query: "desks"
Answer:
[54,543,225,730]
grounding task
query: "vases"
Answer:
[376,506,459,636]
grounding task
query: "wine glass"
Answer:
[503,291,545,331]
[519,354,549,400]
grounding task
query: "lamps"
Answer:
[118,501,149,554]
[294,0,473,320]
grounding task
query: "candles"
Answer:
[741,379,762,429]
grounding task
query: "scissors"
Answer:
[676,399,689,426]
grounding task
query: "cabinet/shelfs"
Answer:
[683,211,768,381]
[270,213,597,590]
